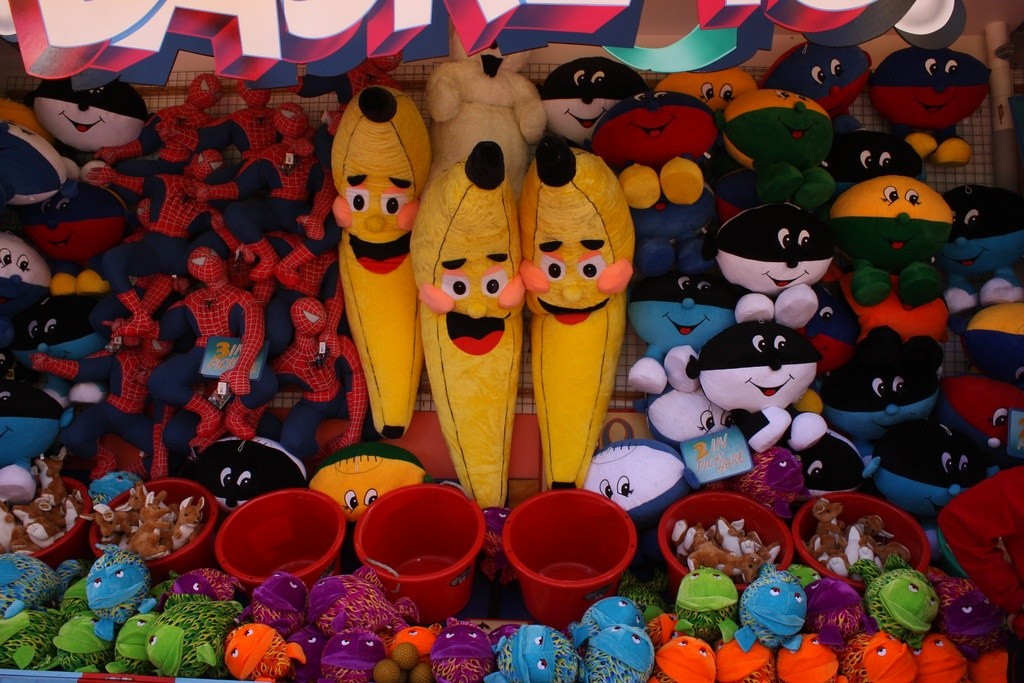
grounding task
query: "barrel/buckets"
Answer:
[791,491,929,595]
[90,478,219,579]
[503,490,638,628]
[355,482,486,623]
[31,475,92,568]
[215,489,347,594]
[658,493,794,596]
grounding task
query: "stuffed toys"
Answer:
[0,58,1024,683]
